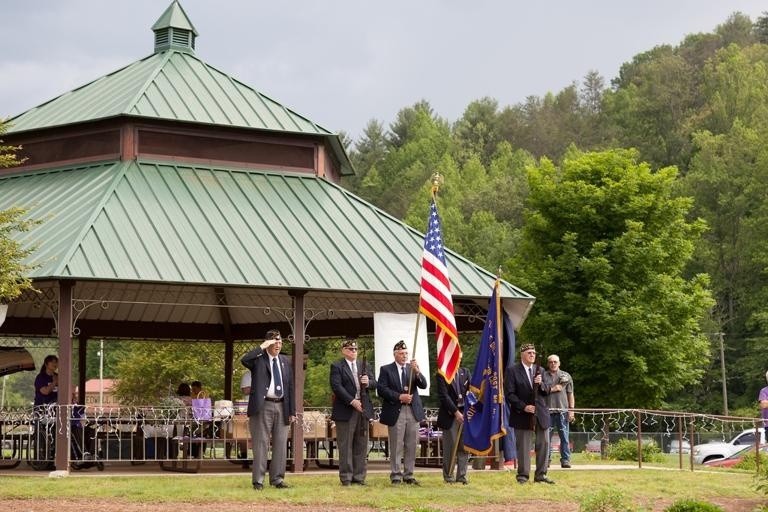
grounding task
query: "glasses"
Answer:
[345,348,357,351]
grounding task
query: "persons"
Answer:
[174,381,193,414]
[71,392,86,427]
[434,346,473,485]
[33,354,59,467]
[502,341,558,486]
[327,338,378,487]
[375,340,429,486]
[757,371,768,447]
[190,380,204,400]
[542,352,576,469]
[240,369,252,397]
[239,330,297,489]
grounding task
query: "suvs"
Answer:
[550,435,573,452]
[688,428,765,466]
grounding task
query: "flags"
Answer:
[461,279,510,455]
[416,198,465,383]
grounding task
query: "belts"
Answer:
[265,398,284,403]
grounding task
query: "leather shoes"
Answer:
[517,464,571,485]
[253,476,468,490]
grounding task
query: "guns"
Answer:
[357,344,371,429]
[531,345,541,418]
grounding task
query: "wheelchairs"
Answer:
[26,402,105,471]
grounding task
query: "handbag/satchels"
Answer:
[214,400,235,421]
[192,391,212,420]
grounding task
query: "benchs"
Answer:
[226,410,390,464]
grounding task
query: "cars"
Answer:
[702,444,768,466]
[700,438,722,443]
[584,440,601,453]
[667,439,690,454]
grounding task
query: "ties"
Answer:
[455,373,461,395]
[352,363,360,398]
[272,358,282,397]
[528,368,533,384]
[401,367,407,394]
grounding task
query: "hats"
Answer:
[520,343,535,352]
[393,340,407,351]
[343,340,357,347]
[265,329,281,340]
